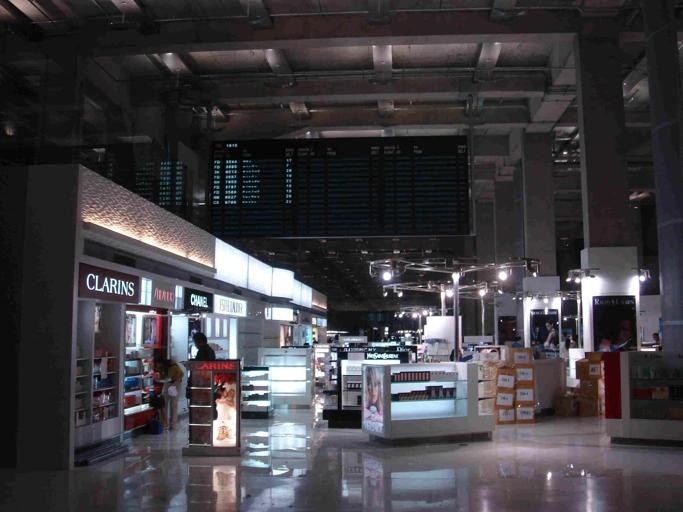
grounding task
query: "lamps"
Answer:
[566,268,652,283]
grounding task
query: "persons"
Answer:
[153,352,183,431]
[450,349,473,362]
[544,319,559,348]
[652,332,660,345]
[362,366,384,423]
[600,314,637,352]
[191,332,215,359]
[214,376,237,447]
[512,336,523,347]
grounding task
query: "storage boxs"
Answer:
[494,347,603,426]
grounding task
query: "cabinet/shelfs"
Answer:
[74,353,121,427]
[123,356,159,417]
[180,345,497,457]
